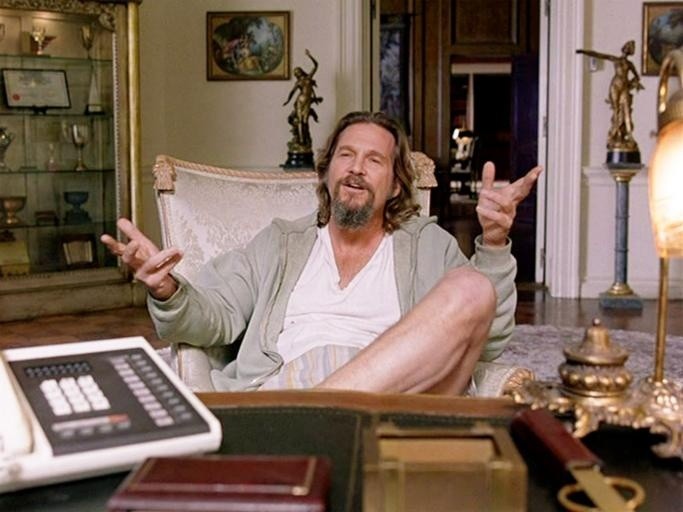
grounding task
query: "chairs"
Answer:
[447,126,478,197]
[149,150,535,396]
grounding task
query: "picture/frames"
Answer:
[639,1,683,78]
[53,231,100,273]
[203,9,291,83]
[0,68,72,109]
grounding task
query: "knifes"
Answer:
[511,406,629,512]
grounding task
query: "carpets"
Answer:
[488,318,683,404]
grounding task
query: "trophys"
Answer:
[0,24,103,227]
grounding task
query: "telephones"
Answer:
[0,336,222,494]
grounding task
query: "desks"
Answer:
[0,388,683,512]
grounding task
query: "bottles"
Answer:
[45,142,58,171]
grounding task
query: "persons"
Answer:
[100,111,543,395]
[576,40,645,140]
[282,49,323,147]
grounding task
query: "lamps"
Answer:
[630,51,683,462]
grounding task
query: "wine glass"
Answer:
[80,23,96,60]
[70,123,89,171]
[62,192,89,213]
[0,196,25,224]
[32,25,46,54]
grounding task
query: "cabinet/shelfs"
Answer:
[0,1,140,295]
